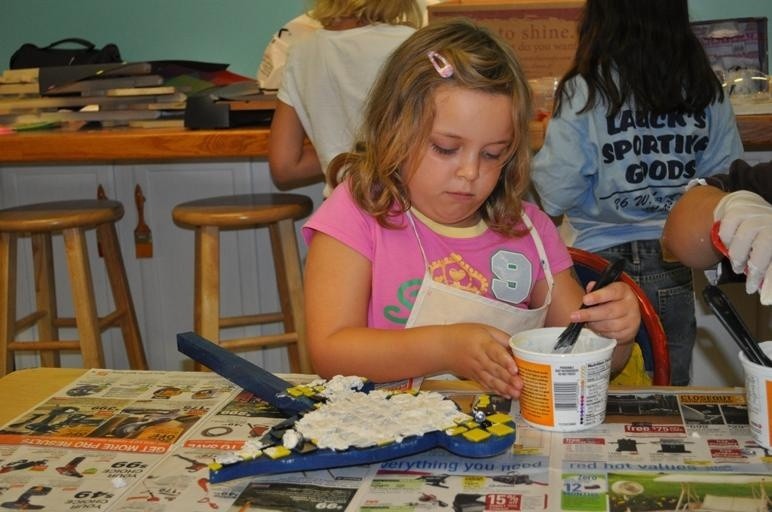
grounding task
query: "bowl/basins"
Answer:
[509,324,617,430]
[736,338,772,453]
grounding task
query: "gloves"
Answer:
[713,190,772,305]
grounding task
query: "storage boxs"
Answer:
[426,1,593,96]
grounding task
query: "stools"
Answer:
[1,201,151,370]
[172,193,317,371]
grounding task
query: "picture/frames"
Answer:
[680,15,769,89]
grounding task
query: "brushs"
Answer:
[134,183,154,260]
[97,184,107,257]
[551,258,627,355]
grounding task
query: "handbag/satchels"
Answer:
[9,38,124,112]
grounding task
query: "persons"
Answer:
[299,15,642,403]
[265,0,429,200]
[661,159,772,308]
[526,1,746,388]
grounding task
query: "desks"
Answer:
[1,366,771,512]
[0,126,335,368]
